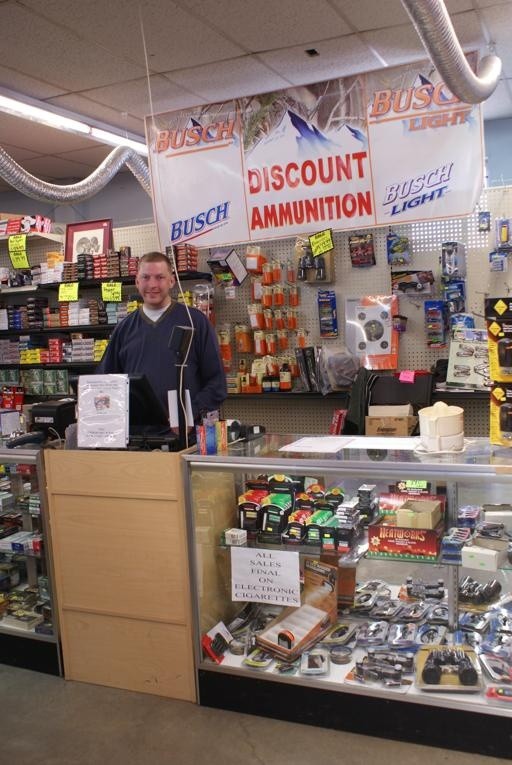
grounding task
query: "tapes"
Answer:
[330,646,352,664]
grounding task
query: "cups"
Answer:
[418,402,464,451]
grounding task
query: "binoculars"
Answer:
[457,576,502,605]
[422,649,478,685]
[297,251,326,281]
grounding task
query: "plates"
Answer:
[415,440,473,456]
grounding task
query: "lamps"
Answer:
[91,126,147,157]
[0,96,91,133]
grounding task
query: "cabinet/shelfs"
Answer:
[210,236,346,396]
[1,267,211,405]
[180,433,512,760]
[0,436,64,677]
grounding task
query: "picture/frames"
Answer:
[64,218,112,261]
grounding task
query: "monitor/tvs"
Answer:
[69,374,170,426]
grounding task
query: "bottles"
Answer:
[280,362,291,392]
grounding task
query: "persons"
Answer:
[92,249,228,426]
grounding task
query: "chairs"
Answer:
[370,374,433,436]
